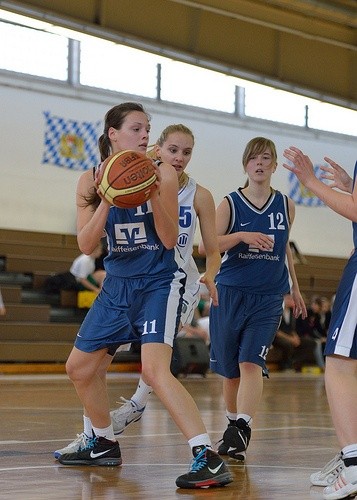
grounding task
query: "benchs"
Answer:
[0,229,349,361]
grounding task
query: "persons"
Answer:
[60,242,106,292]
[181,273,337,373]
[54,123,222,459]
[198,137,308,461]
[282,146,357,500]
[55,101,235,488]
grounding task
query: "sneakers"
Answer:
[215,416,236,456]
[323,464,357,500]
[175,444,233,489]
[310,450,345,487]
[223,417,251,462]
[54,432,93,459]
[109,399,146,435]
[59,435,123,466]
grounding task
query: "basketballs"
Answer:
[97,149,159,209]
